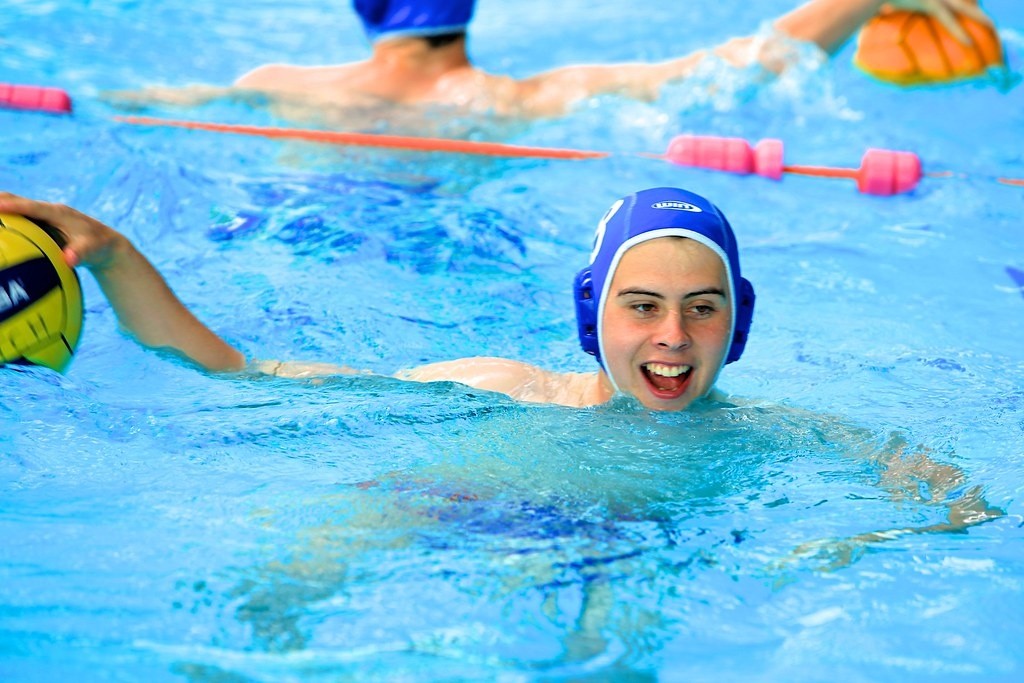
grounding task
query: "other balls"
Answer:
[0,211,84,374]
[853,2,1006,85]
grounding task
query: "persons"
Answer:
[232,0,994,117]
[0,186,755,411]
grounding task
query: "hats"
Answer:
[352,0,476,42]
[573,188,754,367]
